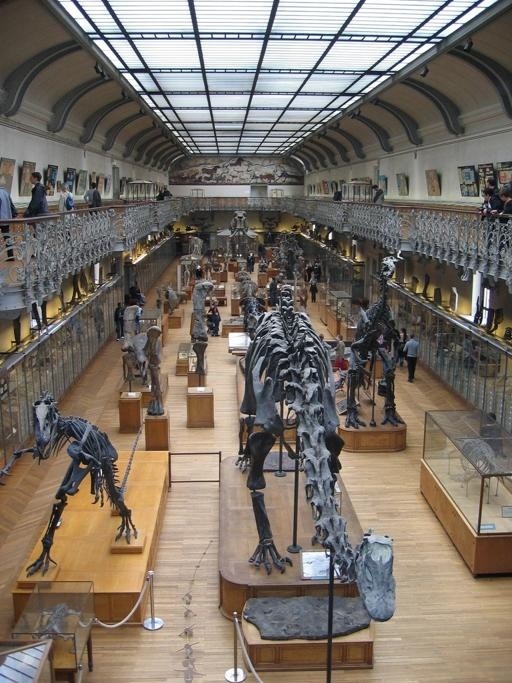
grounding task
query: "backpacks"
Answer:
[65,193,74,211]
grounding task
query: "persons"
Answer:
[371,185,384,204]
[19,171,49,257]
[298,255,321,283]
[247,253,255,273]
[399,327,409,367]
[94,304,106,341]
[150,231,164,246]
[196,265,203,280]
[359,297,369,311]
[333,191,342,201]
[207,304,221,337]
[300,223,337,249]
[403,334,419,383]
[156,190,164,201]
[163,188,172,197]
[309,283,318,303]
[186,225,191,231]
[270,276,282,306]
[83,182,102,214]
[319,333,332,350]
[125,282,145,306]
[335,334,345,372]
[478,175,511,269]
[116,302,125,340]
[478,410,504,460]
[59,182,75,241]
[1,185,18,261]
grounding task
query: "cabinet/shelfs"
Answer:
[117,245,388,450]
[412,404,512,578]
[240,608,374,671]
[11,578,98,682]
